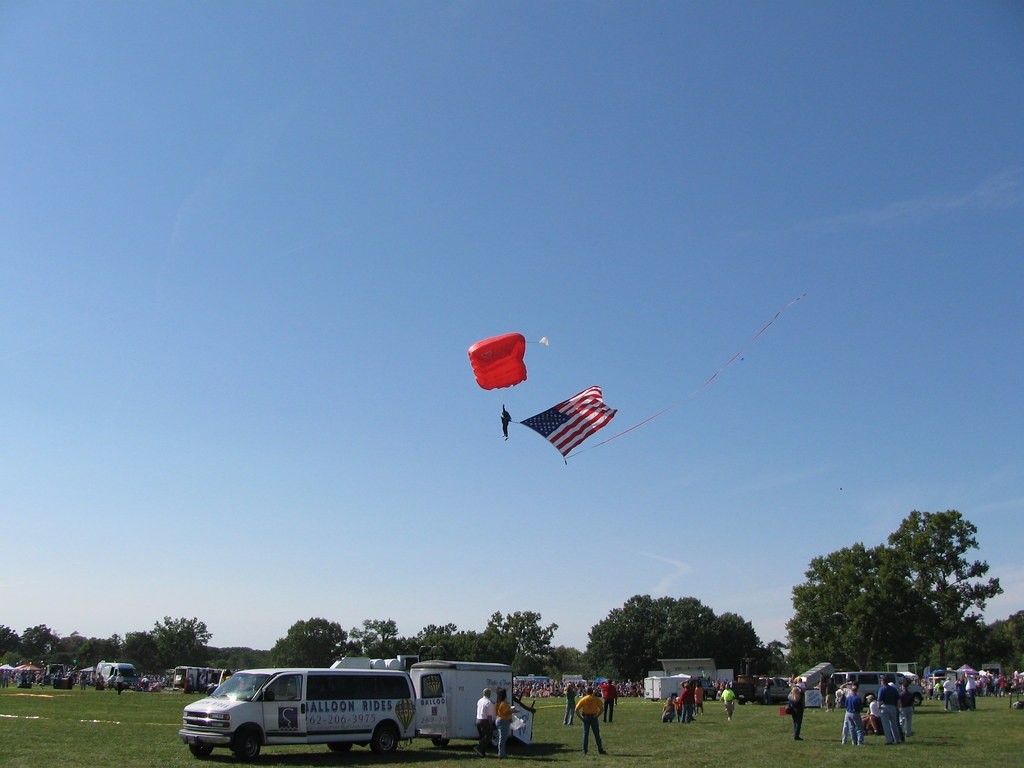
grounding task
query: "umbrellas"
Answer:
[0,663,42,671]
[977,670,994,677]
[956,664,979,675]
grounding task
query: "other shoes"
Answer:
[728,717,731,721]
[795,737,803,740]
[701,713,704,715]
[959,710,961,712]
[857,742,863,745]
[852,742,856,745]
[826,709,830,712]
[474,746,484,758]
[944,709,948,710]
[599,751,606,754]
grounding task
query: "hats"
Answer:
[866,695,874,698]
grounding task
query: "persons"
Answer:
[562,681,577,725]
[839,670,1024,746]
[513,675,645,698]
[661,677,771,723]
[787,668,837,741]
[0,669,45,688]
[602,679,617,723]
[501,403,513,441]
[495,687,520,760]
[473,688,494,758]
[44,667,232,696]
[575,687,606,756]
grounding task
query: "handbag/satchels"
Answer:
[786,706,791,714]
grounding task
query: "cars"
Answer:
[691,677,717,701]
[750,678,792,705]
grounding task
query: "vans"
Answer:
[178,667,418,763]
[829,670,926,706]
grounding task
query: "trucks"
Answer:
[173,665,234,692]
[95,659,139,689]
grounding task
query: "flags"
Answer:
[519,385,618,458]
